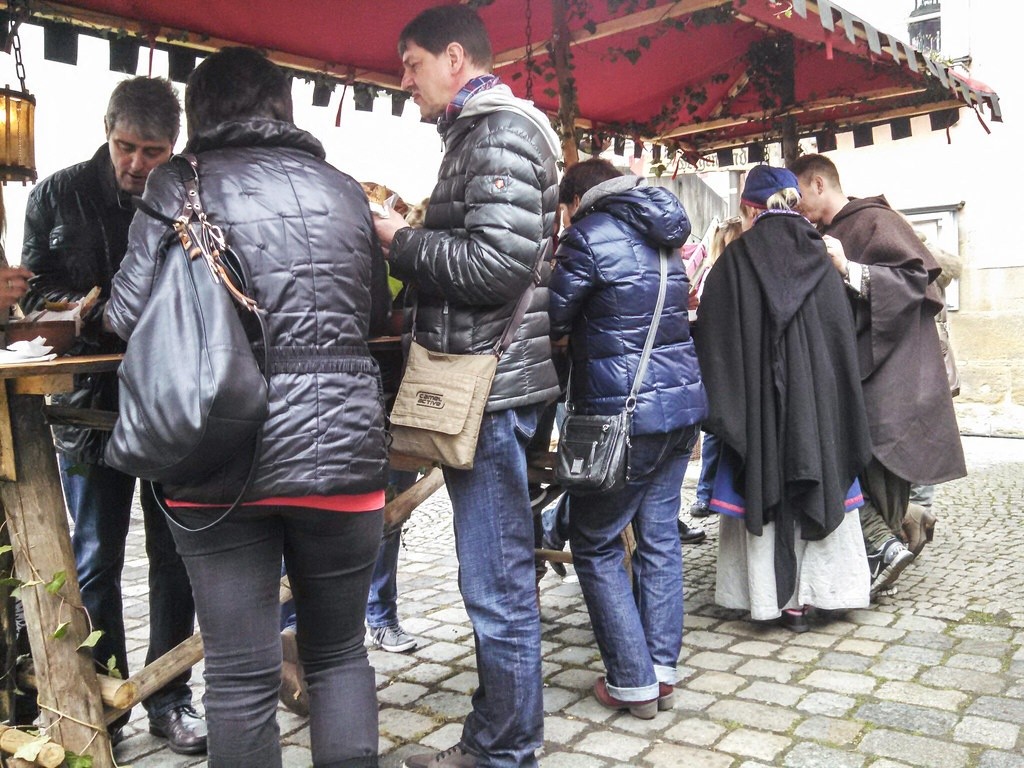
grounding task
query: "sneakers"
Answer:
[405,742,480,768]
[370,625,417,652]
[876,584,898,596]
[868,538,915,603]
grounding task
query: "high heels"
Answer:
[658,682,673,711]
[900,510,934,542]
[903,503,937,558]
[594,676,658,719]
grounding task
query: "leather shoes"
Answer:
[111,728,123,747]
[678,519,706,544]
[149,705,207,754]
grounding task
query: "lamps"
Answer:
[0,83,40,187]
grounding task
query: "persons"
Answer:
[276,181,416,719]
[544,156,709,721]
[540,489,705,578]
[690,215,744,517]
[367,3,565,768]
[0,181,37,328]
[97,44,392,768]
[689,166,873,636]
[19,75,209,753]
[789,152,968,598]
[889,208,962,560]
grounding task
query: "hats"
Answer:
[741,165,802,208]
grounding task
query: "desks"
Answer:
[0,334,636,768]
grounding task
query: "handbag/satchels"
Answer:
[104,154,268,532]
[553,412,632,498]
[387,341,498,469]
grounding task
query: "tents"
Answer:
[0,0,1003,161]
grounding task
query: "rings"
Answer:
[8,280,14,289]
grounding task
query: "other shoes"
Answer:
[815,608,852,618]
[691,506,717,517]
[536,561,548,585]
[779,605,809,633]
[542,538,567,576]
[281,626,310,717]
